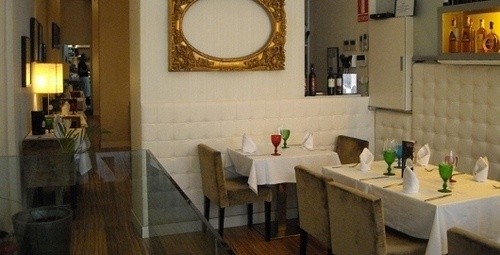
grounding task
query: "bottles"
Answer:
[326,67,336,95]
[309,64,316,96]
[336,68,343,95]
[462,17,476,52]
[482,22,500,53]
[447,19,460,53]
[476,19,487,53]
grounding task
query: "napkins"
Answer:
[243,133,257,153]
[359,147,374,172]
[403,166,419,193]
[473,156,489,182]
[303,132,313,152]
[417,144,430,166]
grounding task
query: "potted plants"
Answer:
[11,123,112,255]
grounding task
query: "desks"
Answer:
[53,111,89,128]
[25,128,92,175]
[323,158,500,255]
[225,144,342,240]
[1,149,235,255]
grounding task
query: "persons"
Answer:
[78,54,91,106]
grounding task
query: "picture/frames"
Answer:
[22,17,60,88]
[168,0,287,72]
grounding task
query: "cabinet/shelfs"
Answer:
[437,0,500,66]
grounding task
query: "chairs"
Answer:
[199,143,272,245]
[20,92,83,207]
[336,136,369,164]
[295,165,500,255]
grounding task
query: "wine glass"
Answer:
[383,138,397,175]
[443,151,458,182]
[393,144,402,169]
[279,129,290,149]
[437,162,453,193]
[271,135,281,156]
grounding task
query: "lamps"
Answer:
[31,62,64,118]
